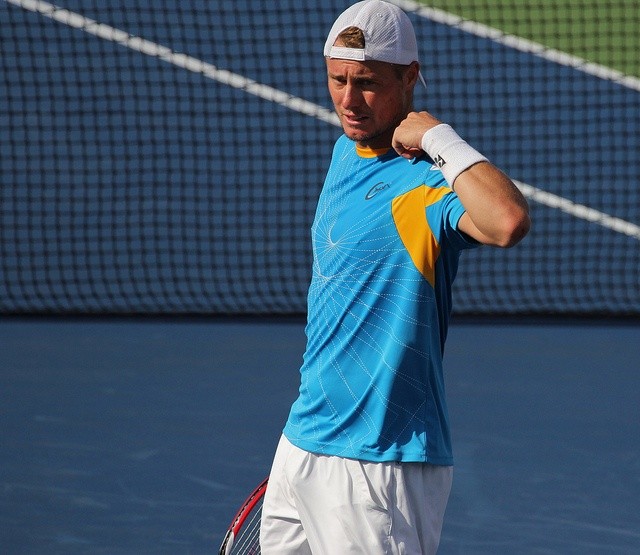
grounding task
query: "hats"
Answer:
[324,0,427,89]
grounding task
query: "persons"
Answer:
[259,0,531,555]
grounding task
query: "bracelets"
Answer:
[421,123,490,192]
